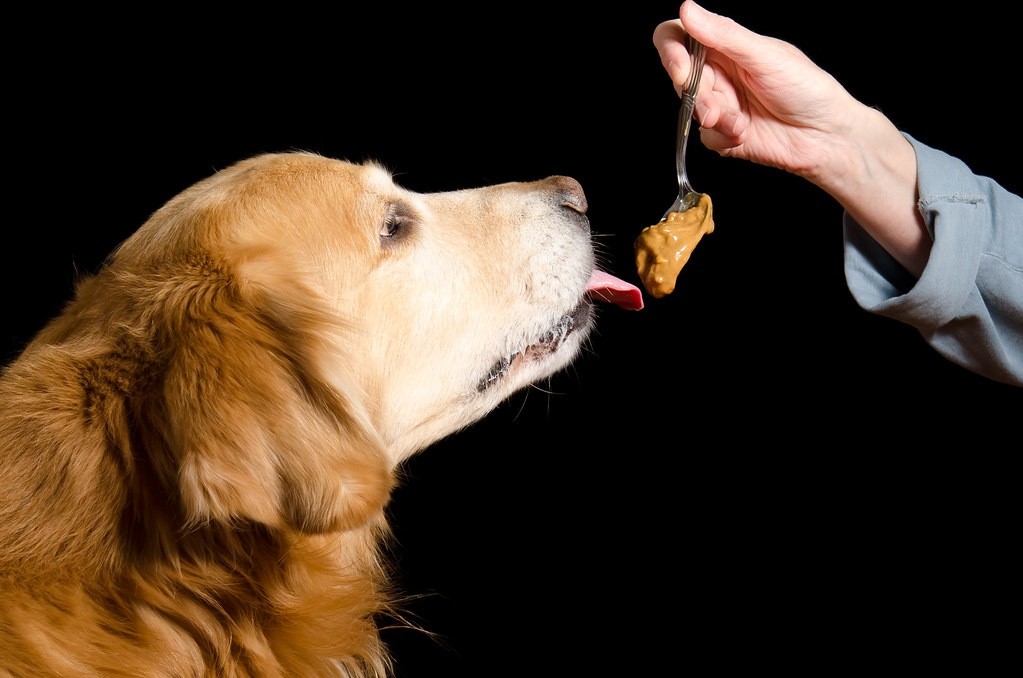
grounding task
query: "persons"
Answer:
[653,0,1023,385]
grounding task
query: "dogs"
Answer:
[0,147,647,678]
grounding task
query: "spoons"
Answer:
[659,29,713,223]
